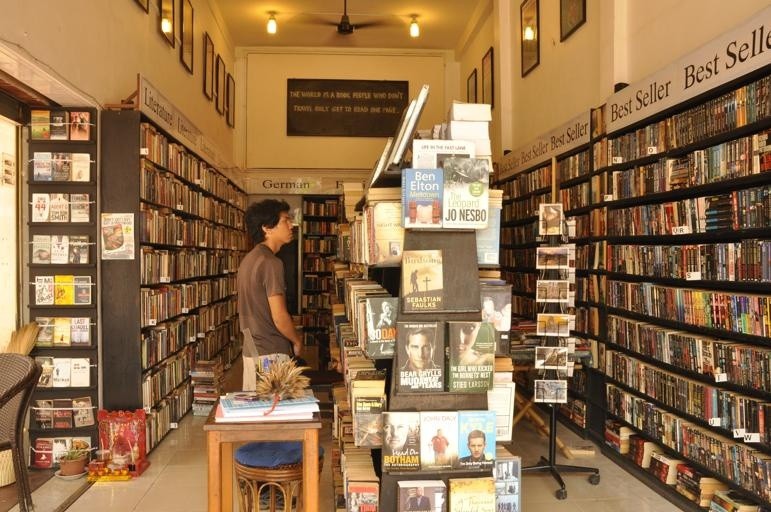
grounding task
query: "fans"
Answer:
[302,0,404,47]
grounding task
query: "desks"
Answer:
[204,396,322,512]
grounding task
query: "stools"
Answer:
[233,438,324,512]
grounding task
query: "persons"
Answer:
[429,430,449,465]
[409,488,431,512]
[237,200,300,382]
[402,331,439,386]
[460,431,490,469]
[385,415,416,456]
[449,324,482,364]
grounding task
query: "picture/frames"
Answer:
[520,0,539,77]
[158,0,175,50]
[226,73,236,127]
[134,0,150,16]
[560,0,586,42]
[179,0,194,75]
[216,53,226,116]
[482,47,494,109]
[203,31,214,102]
[466,67,477,103]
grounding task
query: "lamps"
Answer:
[268,11,276,33]
[409,16,419,38]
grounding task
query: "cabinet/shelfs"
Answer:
[494,101,609,452]
[102,111,250,459]
[299,193,342,378]
[345,173,506,512]
[18,106,100,471]
[602,63,771,512]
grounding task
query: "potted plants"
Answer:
[54,449,88,481]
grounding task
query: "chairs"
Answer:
[0,352,43,512]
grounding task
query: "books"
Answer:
[553,182,589,209]
[558,398,586,429]
[495,164,549,198]
[590,275,770,337]
[403,251,443,312]
[34,355,90,387]
[305,330,329,345]
[503,274,536,294]
[575,244,588,269]
[303,220,339,236]
[142,358,168,409]
[503,193,546,223]
[304,239,332,254]
[478,269,511,359]
[303,199,338,217]
[198,296,238,334]
[33,234,89,264]
[337,180,403,265]
[334,262,398,361]
[174,249,238,282]
[331,301,387,448]
[363,86,429,187]
[486,373,514,442]
[556,150,589,184]
[34,277,93,305]
[174,179,205,218]
[167,348,195,393]
[34,396,95,429]
[607,313,770,392]
[35,317,90,346]
[303,313,332,326]
[410,101,492,172]
[302,275,334,292]
[594,242,770,282]
[30,110,91,142]
[575,280,589,304]
[146,377,193,452]
[492,446,522,512]
[398,480,447,512]
[140,322,170,373]
[566,214,591,238]
[181,275,237,313]
[654,443,770,512]
[139,203,183,245]
[140,157,174,206]
[444,156,488,229]
[214,392,320,422]
[420,411,459,471]
[592,75,770,170]
[140,122,169,169]
[648,369,771,453]
[188,358,225,416]
[204,197,250,231]
[227,185,249,210]
[168,313,198,353]
[605,386,654,470]
[512,323,591,364]
[458,410,496,471]
[140,246,176,284]
[32,151,90,183]
[303,257,332,273]
[512,296,537,319]
[572,309,589,335]
[476,189,502,265]
[198,316,243,369]
[302,294,331,309]
[593,186,770,239]
[221,228,252,251]
[199,160,229,201]
[382,412,421,472]
[401,169,444,226]
[32,194,88,223]
[449,478,496,511]
[168,144,200,185]
[395,323,444,393]
[592,131,769,203]
[503,247,537,271]
[292,316,304,328]
[504,225,540,244]
[141,284,181,329]
[34,438,91,469]
[447,322,494,394]
[183,218,223,248]
[330,384,379,512]
